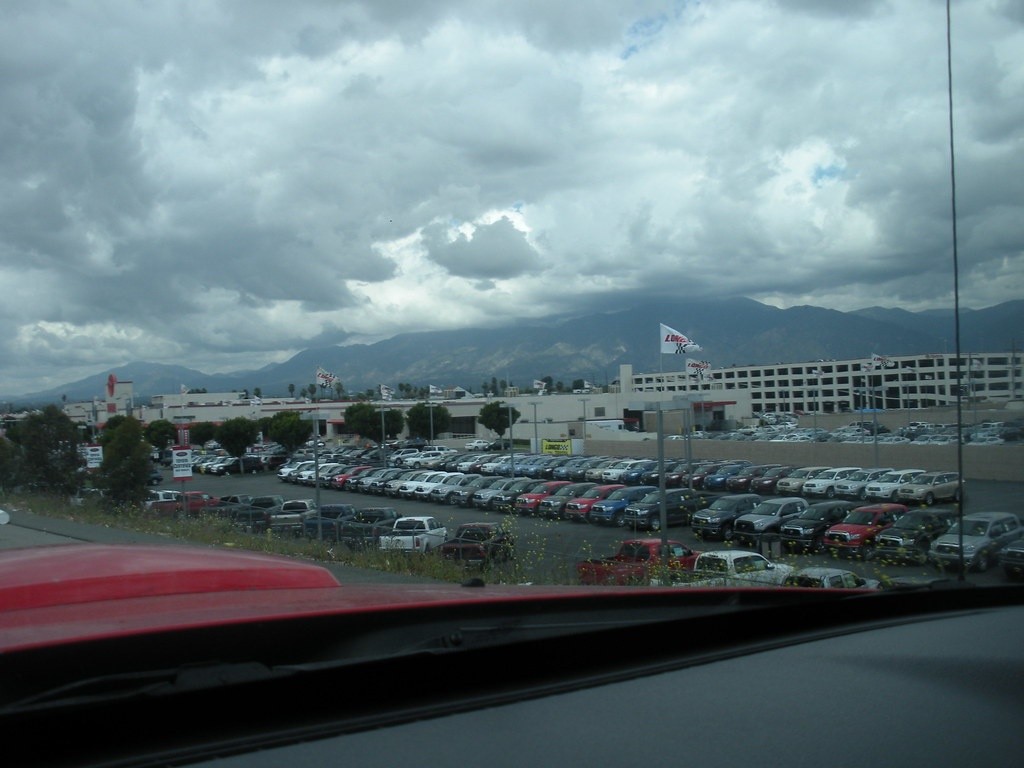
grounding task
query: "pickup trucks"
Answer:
[378,515,446,556]
[270,500,319,531]
[581,538,882,590]
[340,506,404,551]
[304,503,357,539]
[438,522,515,564]
[146,490,282,528]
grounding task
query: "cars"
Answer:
[668,413,829,443]
[834,419,1006,444]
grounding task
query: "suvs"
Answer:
[252,445,414,496]
[190,455,238,474]
[874,510,964,563]
[928,512,1024,573]
[998,536,1024,578]
[835,467,965,506]
[822,503,909,557]
[148,469,163,486]
[400,446,570,518]
[217,456,263,475]
[566,455,854,549]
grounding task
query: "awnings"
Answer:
[327,419,346,425]
[624,418,640,423]
[97,423,106,429]
[691,401,736,407]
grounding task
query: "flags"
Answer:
[316,366,338,389]
[686,358,712,374]
[430,385,443,393]
[381,384,396,401]
[862,362,875,373]
[250,395,262,408]
[812,369,824,378]
[871,353,895,367]
[861,378,866,382]
[181,384,189,394]
[906,365,918,373]
[660,323,703,353]
[305,397,312,404]
[534,379,547,388]
[972,358,982,370]
